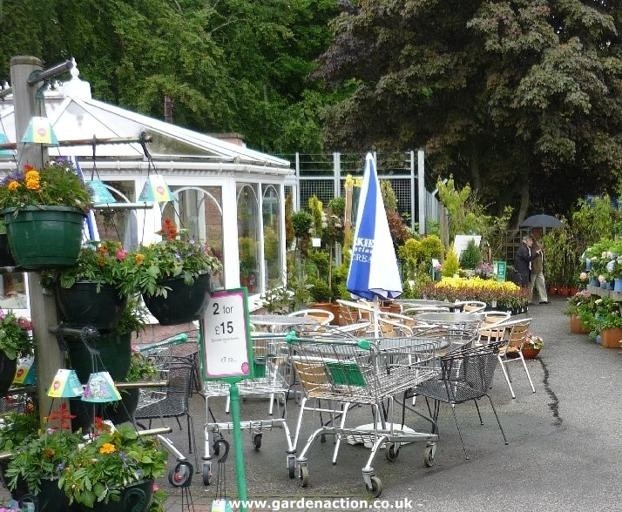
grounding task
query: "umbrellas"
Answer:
[345,152,403,339]
[519,214,565,228]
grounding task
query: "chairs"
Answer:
[287,309,334,331]
[477,318,536,399]
[375,312,429,336]
[455,301,487,314]
[333,321,371,337]
[335,299,374,324]
[470,311,511,344]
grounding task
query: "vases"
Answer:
[600,282,606,289]
[450,304,528,315]
[606,281,613,290]
[585,257,592,271]
[614,278,622,292]
[588,276,599,286]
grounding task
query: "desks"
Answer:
[250,314,317,333]
[416,312,483,337]
[392,298,456,313]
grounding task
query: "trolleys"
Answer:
[135,333,194,487]
[198,332,297,485]
[286,333,439,498]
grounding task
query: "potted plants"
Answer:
[0,306,38,395]
[570,302,591,333]
[81,344,158,424]
[5,405,79,511]
[137,218,218,325]
[41,240,157,332]
[518,332,544,359]
[0,400,41,500]
[58,416,168,512]
[63,295,147,384]
[601,313,622,347]
[0,159,93,269]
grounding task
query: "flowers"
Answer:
[582,247,597,262]
[598,251,622,284]
[579,270,598,281]
[425,275,529,305]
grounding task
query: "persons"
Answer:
[528,227,552,304]
[515,235,541,289]
